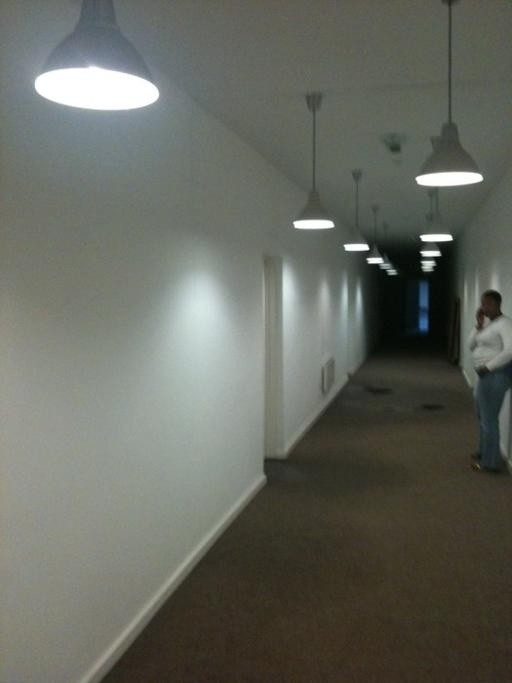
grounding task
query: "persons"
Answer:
[467,290,512,472]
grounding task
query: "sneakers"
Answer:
[471,451,502,472]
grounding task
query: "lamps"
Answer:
[420,242,441,272]
[343,170,370,251]
[415,3,483,188]
[380,223,398,274]
[293,92,335,230]
[31,0,158,114]
[418,136,453,244]
[366,205,384,265]
